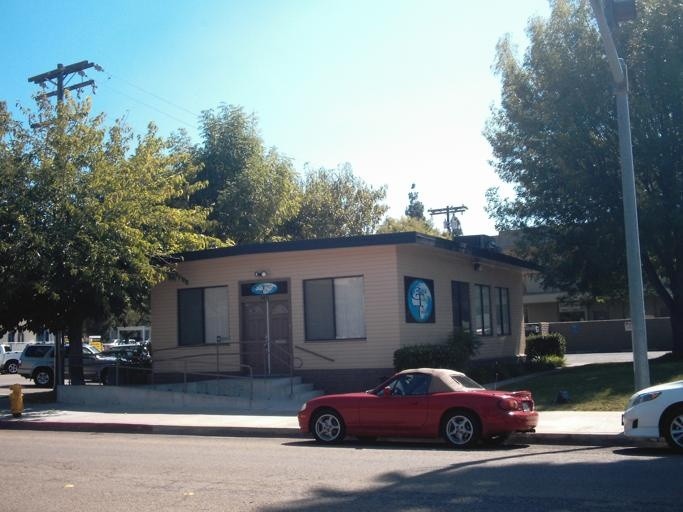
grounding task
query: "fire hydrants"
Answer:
[9,384,24,417]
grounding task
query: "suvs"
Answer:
[16,341,128,388]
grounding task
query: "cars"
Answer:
[620,377,683,455]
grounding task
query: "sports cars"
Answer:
[298,367,540,451]
